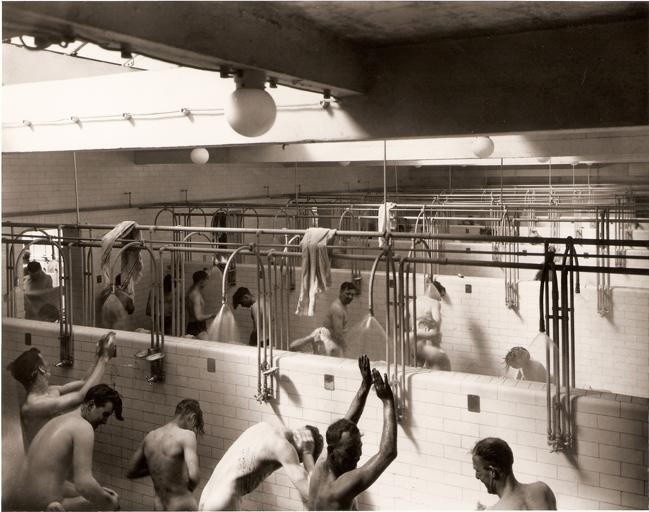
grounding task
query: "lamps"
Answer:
[537,157,551,163]
[471,136,495,159]
[189,148,210,165]
[339,161,350,166]
[224,68,278,138]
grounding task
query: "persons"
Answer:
[33,304,59,324]
[289,328,343,356]
[473,438,556,510]
[9,384,122,511]
[324,282,355,355]
[500,348,547,383]
[306,354,398,509]
[101,275,129,332]
[233,287,270,348]
[148,274,186,336]
[125,397,203,511]
[12,334,118,453]
[186,267,218,341]
[196,420,323,511]
[24,262,52,294]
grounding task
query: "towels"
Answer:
[378,202,396,249]
[101,222,141,291]
[97,332,117,360]
[315,229,336,292]
[295,227,329,317]
